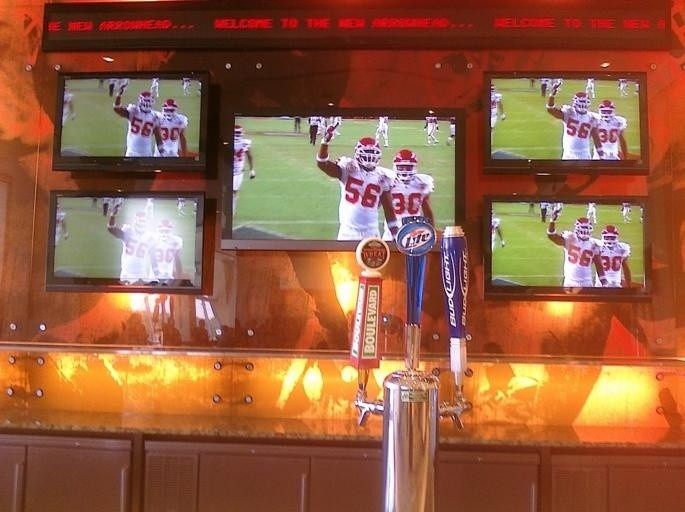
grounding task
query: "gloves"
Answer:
[112,204,119,215]
[502,114,505,119]
[323,122,340,141]
[552,208,562,221]
[552,82,561,95]
[249,171,256,179]
[501,240,505,247]
[116,84,125,95]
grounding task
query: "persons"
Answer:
[490,75,639,161]
[233,114,455,243]
[491,201,644,288]
[55,196,198,287]
[61,76,201,159]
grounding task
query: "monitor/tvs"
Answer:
[46,189,207,296]
[51,69,214,172]
[481,193,653,302]
[482,69,650,177]
[220,103,466,252]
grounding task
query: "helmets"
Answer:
[138,91,154,112]
[574,218,594,240]
[573,92,591,113]
[599,100,616,121]
[354,137,381,169]
[235,124,243,141]
[161,99,179,119]
[601,225,619,248]
[394,150,418,181]
